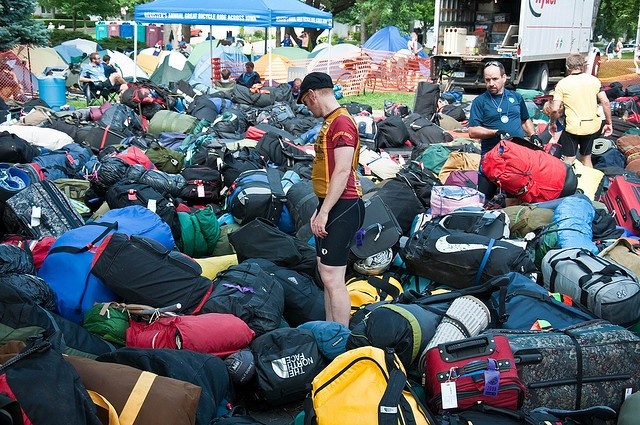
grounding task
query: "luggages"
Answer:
[83,123,126,155]
[424,333,525,407]
[487,317,639,413]
[7,179,85,240]
[603,173,640,234]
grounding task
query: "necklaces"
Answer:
[488,94,506,112]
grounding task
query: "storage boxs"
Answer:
[470,0,509,55]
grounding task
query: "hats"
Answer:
[297,71,334,103]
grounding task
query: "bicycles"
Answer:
[335,58,377,99]
[379,58,411,90]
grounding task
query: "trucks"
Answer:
[429,0,602,93]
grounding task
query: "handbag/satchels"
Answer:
[377,117,409,148]
[355,116,375,149]
[445,170,479,189]
[286,181,319,227]
[396,160,443,207]
[232,84,273,107]
[0,131,36,165]
[174,207,220,258]
[429,185,485,217]
[227,169,295,233]
[304,345,436,424]
[89,158,187,193]
[1,243,56,312]
[350,194,401,259]
[415,81,439,122]
[199,262,284,332]
[438,152,481,185]
[106,181,176,226]
[97,345,228,424]
[343,273,403,319]
[34,142,93,180]
[229,217,318,275]
[297,320,350,360]
[40,205,177,325]
[62,354,202,424]
[147,110,197,134]
[125,312,255,356]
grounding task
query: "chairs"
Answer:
[83,72,120,107]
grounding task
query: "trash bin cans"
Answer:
[137,22,146,43]
[159,25,164,41]
[146,24,159,47]
[96,22,108,41]
[120,22,134,40]
[108,22,120,38]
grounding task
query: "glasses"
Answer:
[485,60,503,67]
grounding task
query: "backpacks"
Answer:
[251,326,320,407]
[121,77,178,119]
[1,303,118,357]
[181,165,223,202]
[414,145,451,172]
[541,247,638,328]
[256,131,284,165]
[363,297,459,368]
[403,225,536,293]
[481,137,578,202]
[487,271,586,332]
[145,143,183,174]
[3,341,102,424]
[248,100,296,128]
[275,114,319,136]
[89,232,212,315]
[403,113,453,149]
[274,82,297,114]
[273,267,326,322]
[97,103,143,138]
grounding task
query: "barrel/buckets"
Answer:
[38,76,66,108]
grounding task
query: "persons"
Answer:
[605,38,616,62]
[240,64,258,84]
[280,33,293,47]
[79,52,128,94]
[547,55,612,166]
[102,54,120,77]
[297,72,364,326]
[219,69,233,84]
[297,30,310,51]
[405,32,420,91]
[177,42,194,57]
[616,37,623,59]
[468,61,534,196]
[153,42,160,56]
[288,78,302,97]
[1,63,28,102]
[206,32,214,39]
